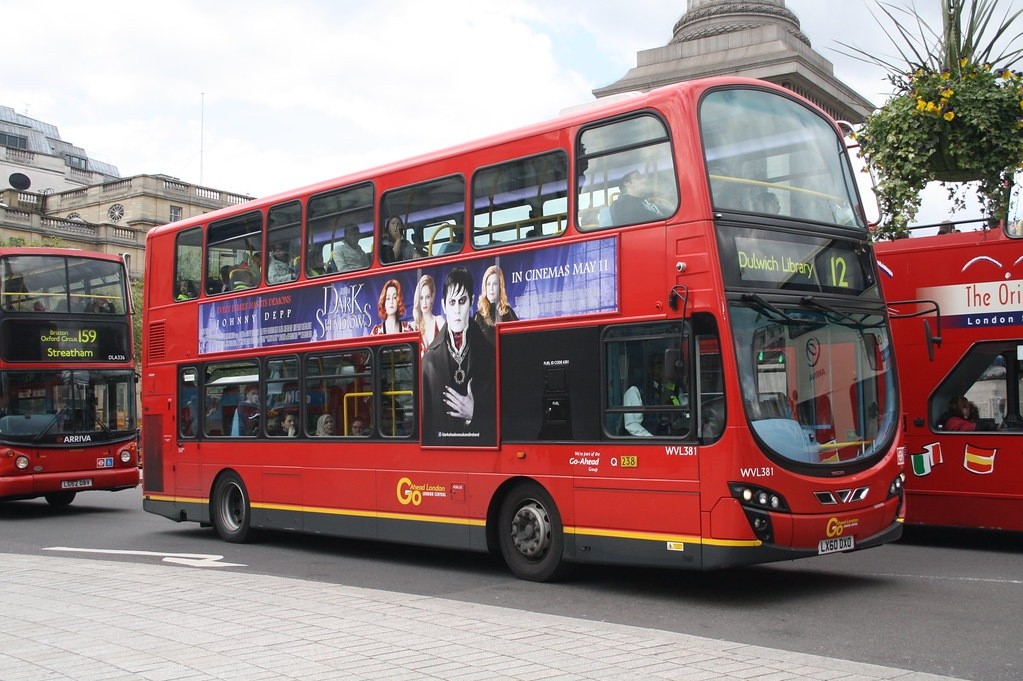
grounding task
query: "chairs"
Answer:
[201,224,507,293]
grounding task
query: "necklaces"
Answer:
[447,327,467,384]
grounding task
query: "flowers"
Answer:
[861,24,1023,227]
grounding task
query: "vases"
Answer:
[927,146,983,181]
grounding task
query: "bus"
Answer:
[140,74,944,583]
[690,227,1023,532]
[0,248,140,508]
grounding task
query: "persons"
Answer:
[526,230,542,238]
[54,290,85,312]
[422,265,497,447]
[473,265,518,349]
[231,383,262,436]
[609,170,668,226]
[34,301,48,310]
[619,376,690,439]
[315,414,334,436]
[276,414,298,437]
[220,252,262,292]
[369,279,414,336]
[410,274,445,358]
[943,395,997,432]
[177,279,189,299]
[217,385,240,435]
[282,379,405,435]
[83,294,115,313]
[703,372,759,444]
[348,417,366,436]
[382,215,422,263]
[0,392,20,418]
[333,223,370,272]
[267,243,297,283]
[79,299,86,308]
[754,192,782,214]
